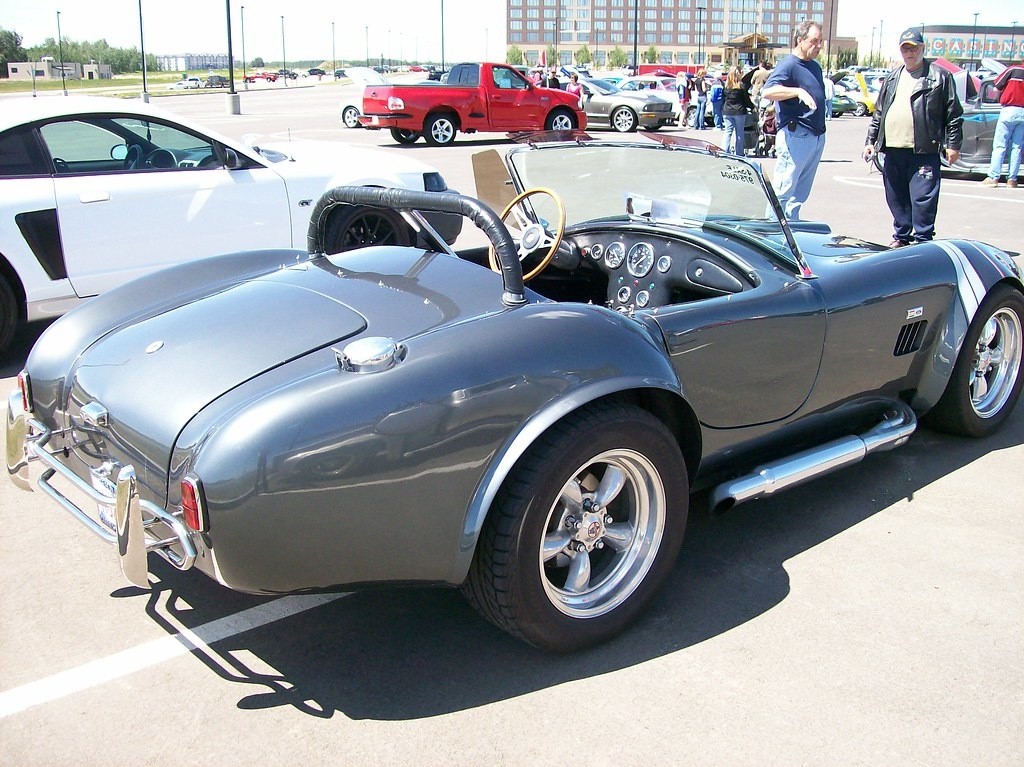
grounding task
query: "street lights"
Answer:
[331,22,336,77]
[870,19,884,67]
[241,5,245,78]
[697,7,706,62]
[57,11,65,90]
[970,12,979,71]
[365,26,369,67]
[281,15,286,83]
[1010,20,1017,66]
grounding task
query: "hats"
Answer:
[898,29,923,46]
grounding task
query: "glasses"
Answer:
[551,72,555,73]
[900,44,922,53]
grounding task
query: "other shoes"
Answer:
[889,240,905,248]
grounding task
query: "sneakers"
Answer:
[980,178,998,187]
[1006,179,1018,188]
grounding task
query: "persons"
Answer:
[566,72,585,110]
[694,70,723,130]
[677,72,694,128]
[824,75,835,121]
[724,66,756,156]
[863,29,964,248]
[532,71,544,81]
[540,70,561,89]
[762,20,826,220]
[982,57,1024,188]
[763,106,777,158]
[750,61,772,118]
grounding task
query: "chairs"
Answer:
[640,84,644,89]
[650,84,656,89]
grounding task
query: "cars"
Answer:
[0,97,462,373]
[6,128,1024,655]
[169,65,448,90]
[511,57,1024,180]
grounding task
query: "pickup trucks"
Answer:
[357,62,588,147]
